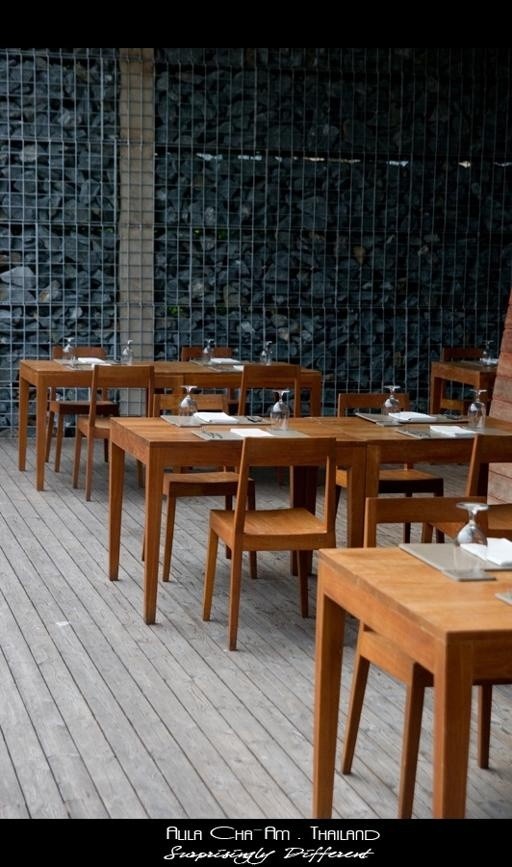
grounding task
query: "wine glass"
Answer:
[468,388,487,429]
[259,340,274,366]
[178,385,199,425]
[384,384,401,422]
[200,338,215,367]
[61,337,76,367]
[480,340,494,367]
[269,389,292,432]
[120,339,134,366]
[453,500,490,568]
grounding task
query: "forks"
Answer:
[201,425,225,441]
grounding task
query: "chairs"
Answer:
[47,340,512,819]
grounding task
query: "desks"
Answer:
[17,357,323,491]
